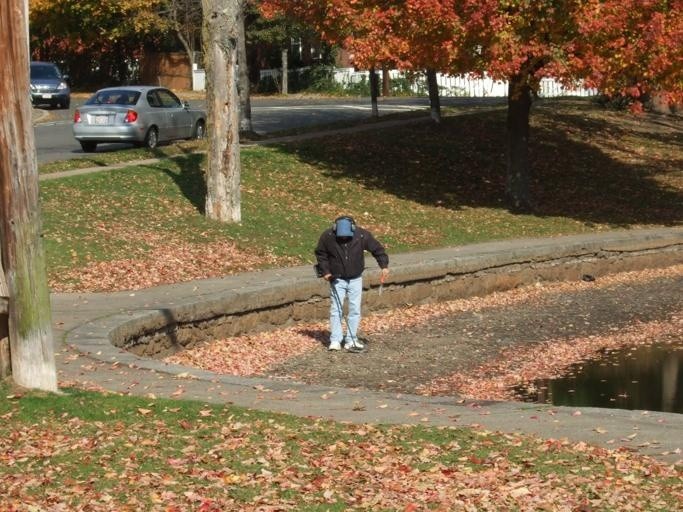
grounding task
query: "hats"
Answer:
[335,220,353,237]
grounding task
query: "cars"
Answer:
[72,84,207,154]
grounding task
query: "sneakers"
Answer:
[343,341,364,350]
[328,341,341,351]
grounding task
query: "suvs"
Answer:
[28,60,73,111]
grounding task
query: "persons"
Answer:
[313,215,390,352]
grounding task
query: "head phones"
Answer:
[332,215,356,233]
